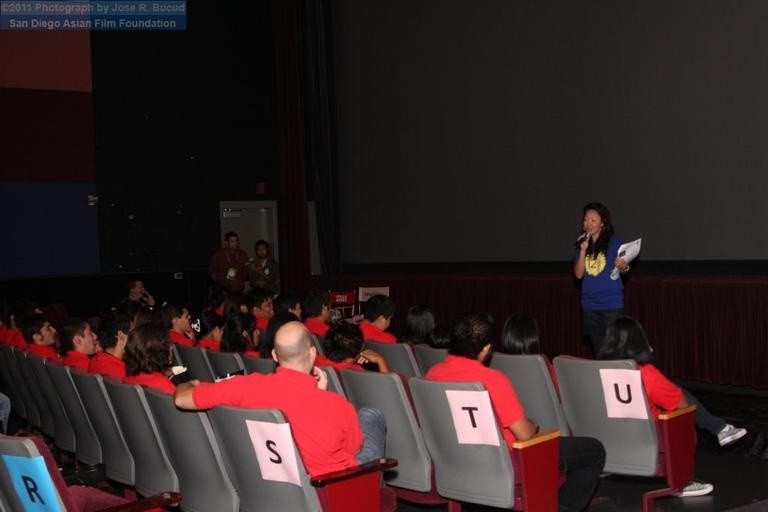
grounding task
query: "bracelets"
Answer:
[620,266,630,272]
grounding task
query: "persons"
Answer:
[422,309,607,512]
[574,200,631,359]
[359,294,559,393]
[246,240,282,296]
[594,315,749,497]
[173,319,387,491]
[194,277,398,372]
[2,279,195,396]
[207,231,251,294]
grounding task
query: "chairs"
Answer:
[326,288,358,318]
[356,285,391,316]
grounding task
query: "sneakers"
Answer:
[677,483,713,497]
[717,424,746,447]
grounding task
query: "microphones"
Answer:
[573,230,594,249]
